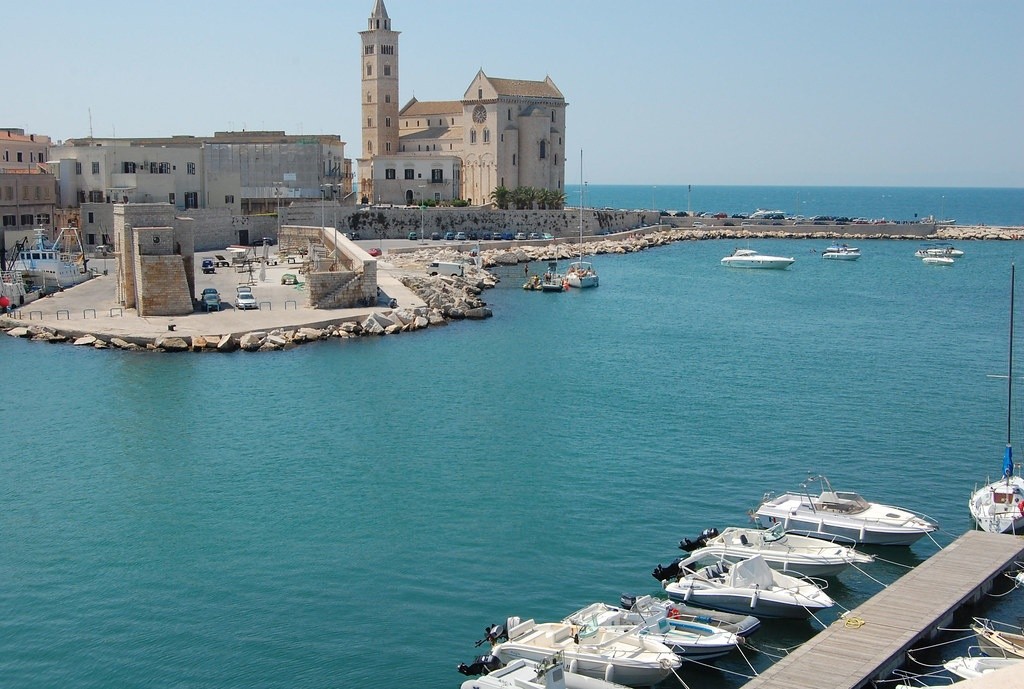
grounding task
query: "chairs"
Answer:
[740,534,754,547]
[704,560,733,583]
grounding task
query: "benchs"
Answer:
[570,603,621,626]
[508,618,546,645]
[552,625,576,647]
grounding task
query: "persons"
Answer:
[524,264,528,277]
[730,248,737,257]
[945,247,952,254]
[545,269,551,284]
[809,249,816,253]
[944,255,949,258]
[1015,498,1024,517]
[919,251,929,255]
[534,275,539,285]
[567,265,590,277]
[471,251,475,257]
[836,242,848,254]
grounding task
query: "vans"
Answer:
[425,262,464,278]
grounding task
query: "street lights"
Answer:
[417,184,427,243]
[275,187,280,233]
[320,189,326,245]
[332,190,338,268]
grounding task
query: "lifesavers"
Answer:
[1017,500,1024,516]
[668,608,680,620]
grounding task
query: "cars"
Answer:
[454,232,466,240]
[541,233,555,239]
[481,232,539,240]
[408,231,417,240]
[430,232,440,240]
[368,247,382,256]
[469,232,478,240]
[253,237,274,246]
[235,291,257,310]
[660,210,922,227]
[446,232,455,240]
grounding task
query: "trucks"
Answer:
[201,259,216,273]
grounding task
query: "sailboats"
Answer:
[968,261,1023,533]
[566,148,600,288]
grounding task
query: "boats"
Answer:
[540,261,565,290]
[559,601,745,664]
[678,525,878,578]
[456,655,634,689]
[747,473,943,547]
[893,560,1024,689]
[619,592,761,639]
[200,288,222,311]
[652,550,837,620]
[4,216,95,296]
[914,243,943,258]
[821,250,862,261]
[473,615,685,688]
[927,242,964,258]
[921,255,955,266]
[0,270,45,314]
[721,249,797,270]
[826,241,859,253]
